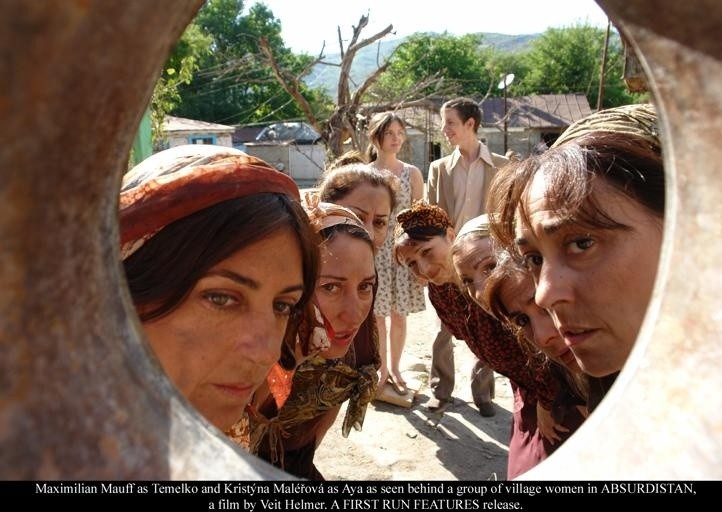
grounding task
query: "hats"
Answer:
[546,101,662,166]
[301,201,370,235]
[118,143,303,266]
[391,199,452,242]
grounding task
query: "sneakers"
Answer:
[428,395,453,414]
[474,401,497,418]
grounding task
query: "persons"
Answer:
[120,97,665,480]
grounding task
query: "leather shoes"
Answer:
[388,369,407,394]
